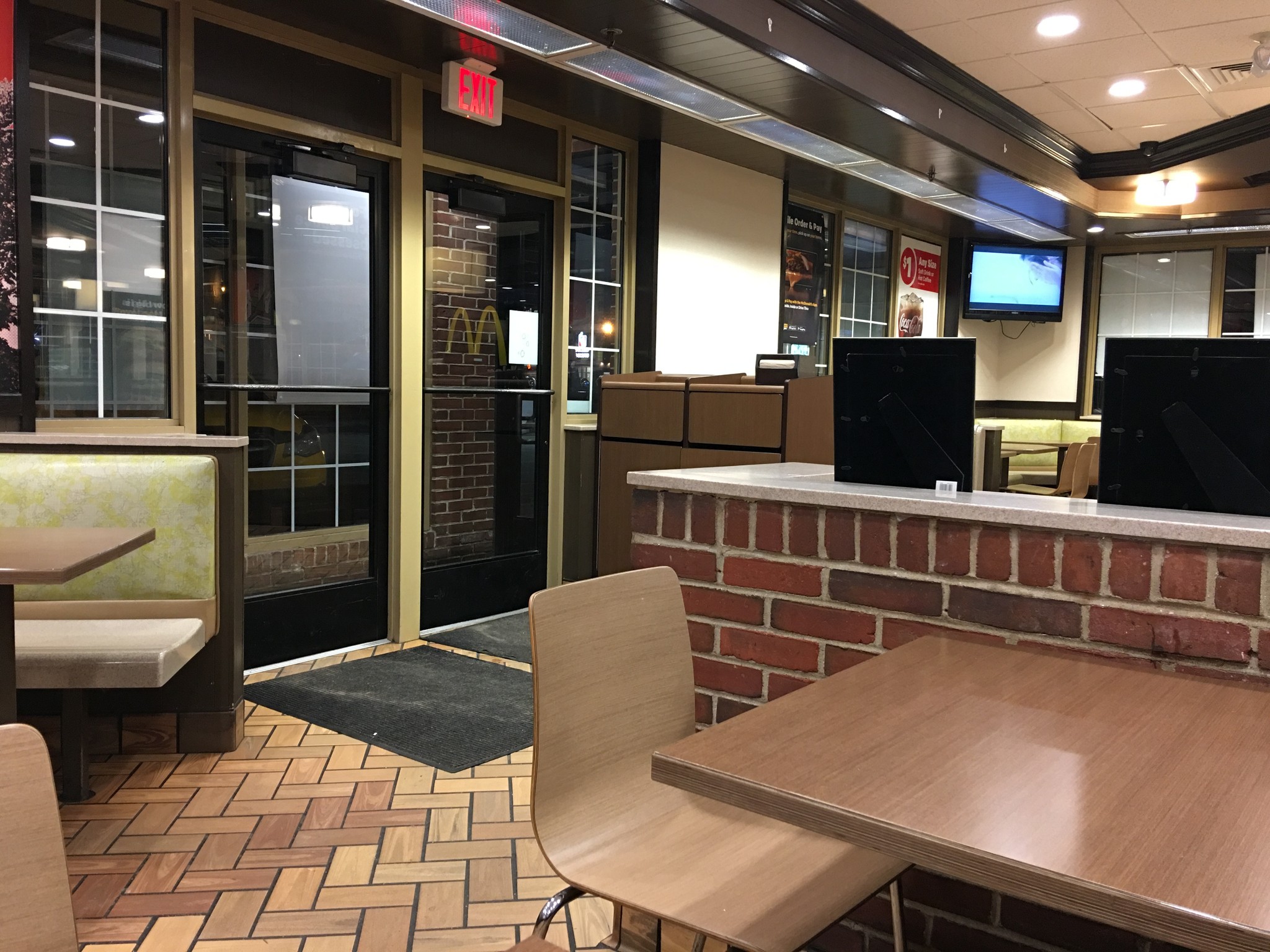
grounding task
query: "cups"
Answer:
[898,296,923,338]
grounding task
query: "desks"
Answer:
[650,634,1270,952]
[0,526,156,728]
[1000,440,1088,487]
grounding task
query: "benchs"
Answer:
[0,453,222,803]
[974,417,1101,471]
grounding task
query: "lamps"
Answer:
[1134,171,1196,206]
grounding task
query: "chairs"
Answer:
[529,565,917,952]
[999,436,1101,498]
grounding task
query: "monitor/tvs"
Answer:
[962,242,1067,322]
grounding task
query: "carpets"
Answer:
[419,610,533,665]
[244,644,533,774]
